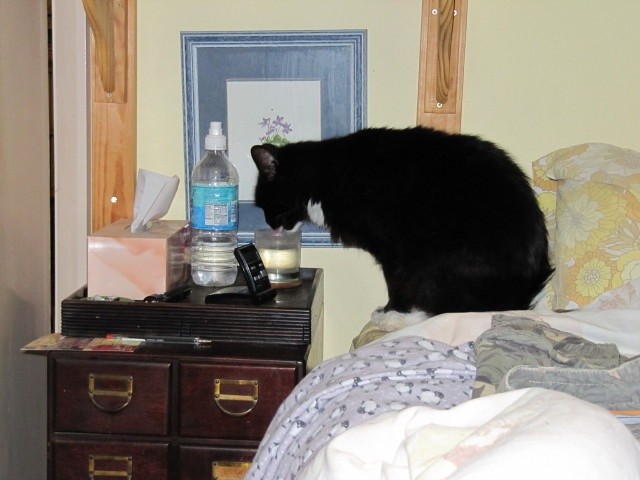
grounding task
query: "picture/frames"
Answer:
[179,29,369,248]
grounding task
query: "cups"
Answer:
[254,227,302,284]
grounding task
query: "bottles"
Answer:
[189,120,239,287]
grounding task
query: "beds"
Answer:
[248,307,640,479]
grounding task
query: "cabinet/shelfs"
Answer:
[48,269,324,480]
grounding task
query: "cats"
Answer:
[250,124,556,331]
